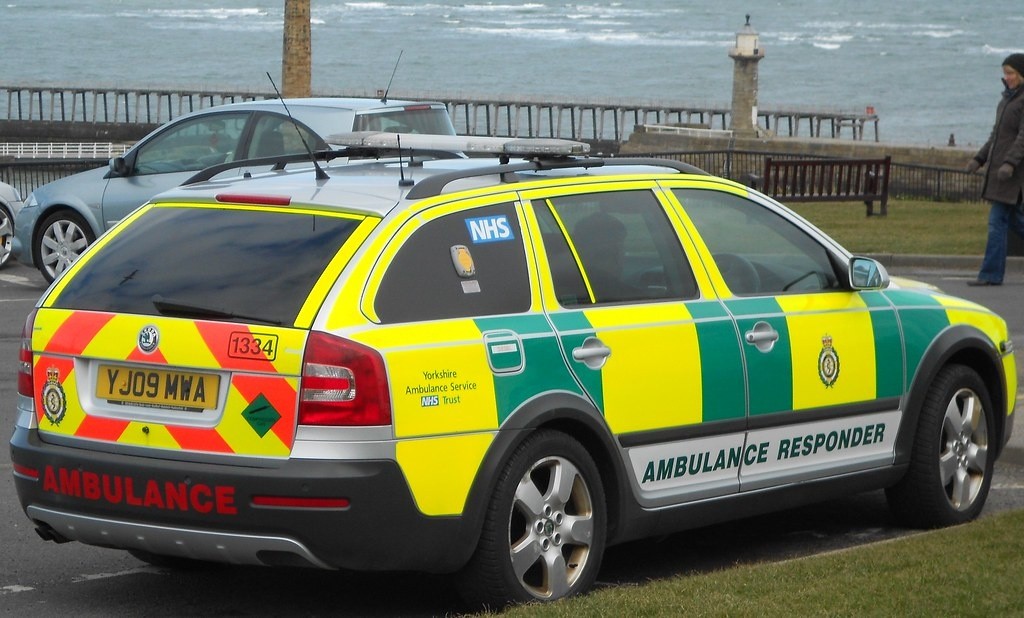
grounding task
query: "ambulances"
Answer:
[8,132,1021,612]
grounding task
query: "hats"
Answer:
[1002,53,1024,78]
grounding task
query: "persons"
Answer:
[964,53,1024,286]
[573,212,627,279]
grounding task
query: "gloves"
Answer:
[998,163,1014,181]
[965,159,981,173]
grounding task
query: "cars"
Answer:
[7,95,466,292]
[0,181,24,271]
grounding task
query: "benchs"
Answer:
[745,154,892,217]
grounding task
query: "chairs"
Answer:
[255,131,285,158]
[710,253,762,293]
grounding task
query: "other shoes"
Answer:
[967,279,1003,286]
